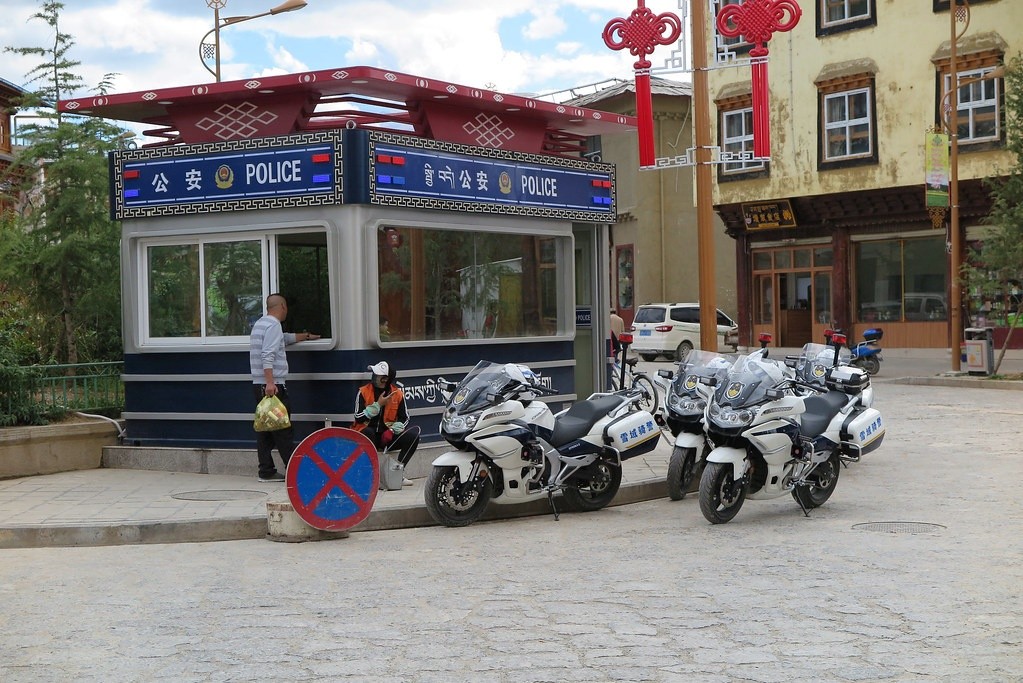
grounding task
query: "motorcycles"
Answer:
[424,333,661,528]
[658,334,797,501]
[694,334,887,526]
[784,329,857,397]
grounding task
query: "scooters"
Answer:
[842,328,884,374]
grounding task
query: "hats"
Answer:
[368,360,396,380]
[610,308,616,311]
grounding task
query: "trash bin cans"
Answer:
[964,326,994,375]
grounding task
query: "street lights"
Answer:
[940,66,1012,372]
[198,0,309,82]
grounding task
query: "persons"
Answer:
[379,317,389,342]
[250,293,321,482]
[610,308,625,364]
[352,361,421,486]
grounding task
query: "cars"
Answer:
[899,292,948,321]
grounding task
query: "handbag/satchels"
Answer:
[253,395,291,432]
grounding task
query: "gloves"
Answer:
[382,430,394,443]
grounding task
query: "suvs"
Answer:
[630,302,738,362]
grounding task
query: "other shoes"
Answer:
[258,472,285,482]
[402,477,414,486]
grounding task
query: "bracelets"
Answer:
[307,333,310,340]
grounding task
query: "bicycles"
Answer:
[611,349,658,416]
